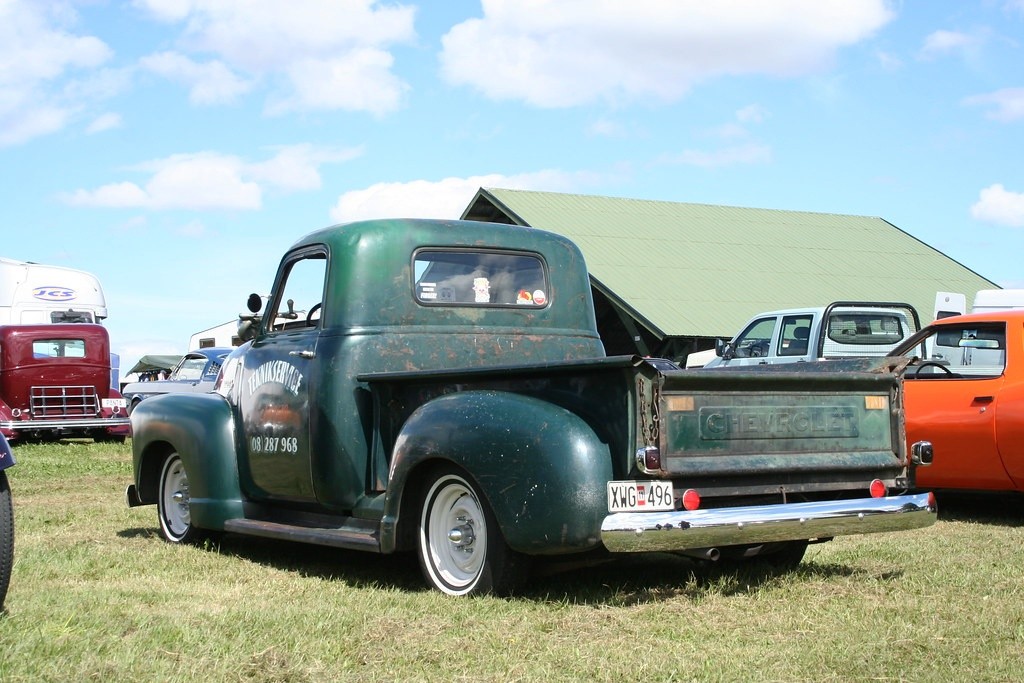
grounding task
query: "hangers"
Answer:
[142,368,159,374]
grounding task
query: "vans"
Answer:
[934,289,1024,321]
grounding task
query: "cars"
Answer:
[122,347,236,416]
[882,311,1024,492]
[0,325,133,444]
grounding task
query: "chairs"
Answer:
[785,327,809,349]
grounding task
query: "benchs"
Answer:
[830,332,901,343]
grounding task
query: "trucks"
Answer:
[0,257,120,392]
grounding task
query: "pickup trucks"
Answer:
[120,218,938,597]
[702,301,1005,376]
